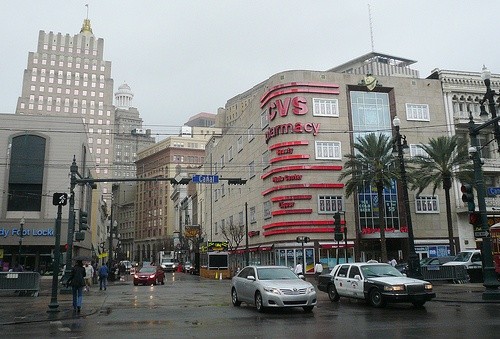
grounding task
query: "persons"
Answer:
[11,260,24,293]
[60,264,68,289]
[313,262,323,284]
[391,257,397,268]
[295,263,303,275]
[113,261,127,282]
[70,259,109,313]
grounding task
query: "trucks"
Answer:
[156,250,176,271]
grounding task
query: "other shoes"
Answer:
[74,306,81,313]
[99,288,102,290]
[104,288,106,290]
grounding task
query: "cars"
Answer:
[183,261,193,272]
[396,249,496,281]
[134,266,165,287]
[230,265,317,313]
[119,260,133,273]
[318,262,436,308]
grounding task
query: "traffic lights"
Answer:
[461,184,476,212]
[469,212,481,226]
[333,212,341,232]
[78,208,88,231]
[52,192,68,206]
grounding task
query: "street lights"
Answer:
[17,216,25,263]
[392,114,424,277]
[479,64,500,149]
